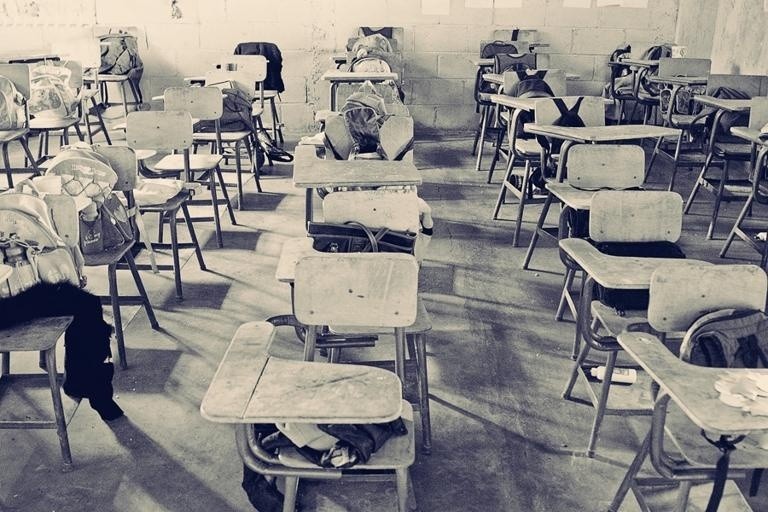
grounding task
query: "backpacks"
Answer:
[680,308,768,368]
[530,97,596,190]
[509,69,557,139]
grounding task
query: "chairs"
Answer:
[1,26,149,191]
[198,26,433,512]
[2,42,286,475]
[469,41,768,510]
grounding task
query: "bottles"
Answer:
[590,366,637,384]
[5,246,37,297]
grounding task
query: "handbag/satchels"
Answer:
[346,34,394,72]
[199,88,253,133]
[707,87,752,144]
[100,33,143,76]
[275,423,408,469]
[609,47,635,100]
[29,65,79,119]
[475,43,518,106]
[307,221,416,253]
[0,190,88,299]
[323,91,388,161]
[235,42,285,94]
[595,240,685,311]
[661,74,708,115]
[0,75,29,131]
[636,45,672,105]
[44,147,134,255]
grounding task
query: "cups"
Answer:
[32,175,62,195]
